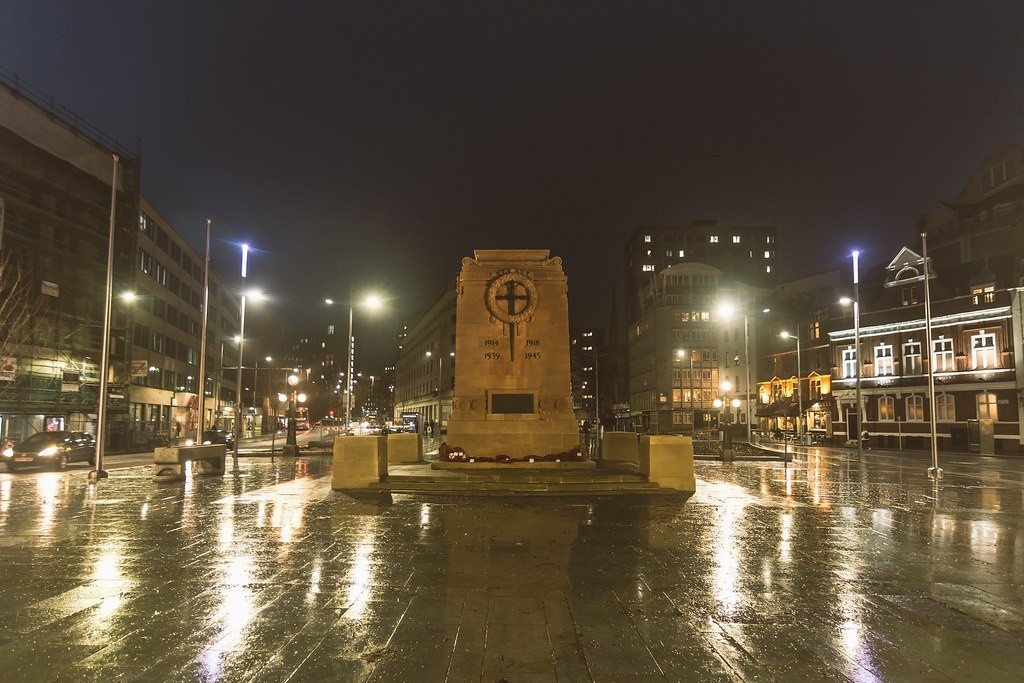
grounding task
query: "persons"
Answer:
[175,422,181,437]
[430,419,435,437]
[583,418,588,437]
[751,411,828,441]
[586,422,592,437]
[211,424,217,429]
[424,418,430,434]
[396,420,411,433]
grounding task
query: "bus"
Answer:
[293,406,311,431]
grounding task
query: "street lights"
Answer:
[920,231,945,474]
[197,216,210,476]
[779,332,806,445]
[234,241,252,461]
[718,305,753,442]
[346,296,382,439]
[87,148,125,479]
[281,375,300,457]
[848,250,868,466]
[323,297,354,437]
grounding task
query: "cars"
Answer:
[3,431,98,473]
[186,430,236,454]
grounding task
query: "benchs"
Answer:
[153,444,226,482]
